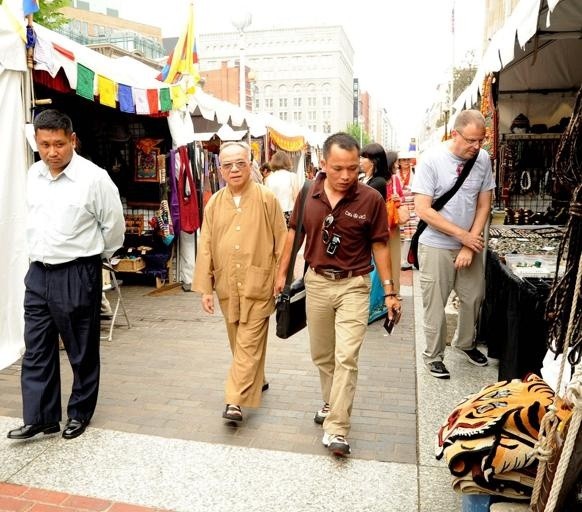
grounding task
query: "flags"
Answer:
[155,4,202,83]
[51,41,190,118]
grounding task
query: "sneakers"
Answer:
[452,341,487,367]
[314,399,332,424]
[423,352,450,378]
[320,431,350,457]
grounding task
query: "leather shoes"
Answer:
[64,413,92,438]
[7,420,60,438]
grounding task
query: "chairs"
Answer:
[102,254,130,344]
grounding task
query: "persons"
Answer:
[268,130,404,457]
[190,139,288,426]
[412,106,497,380]
[251,143,418,326]
[6,109,129,442]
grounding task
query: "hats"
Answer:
[397,151,411,160]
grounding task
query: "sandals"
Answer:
[223,402,242,422]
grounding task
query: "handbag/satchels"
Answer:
[385,172,411,226]
[276,279,311,339]
[409,209,428,269]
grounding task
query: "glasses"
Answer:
[321,213,334,244]
[454,128,484,144]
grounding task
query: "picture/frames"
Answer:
[133,147,163,183]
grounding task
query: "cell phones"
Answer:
[325,233,341,257]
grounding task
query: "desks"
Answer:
[481,218,575,386]
